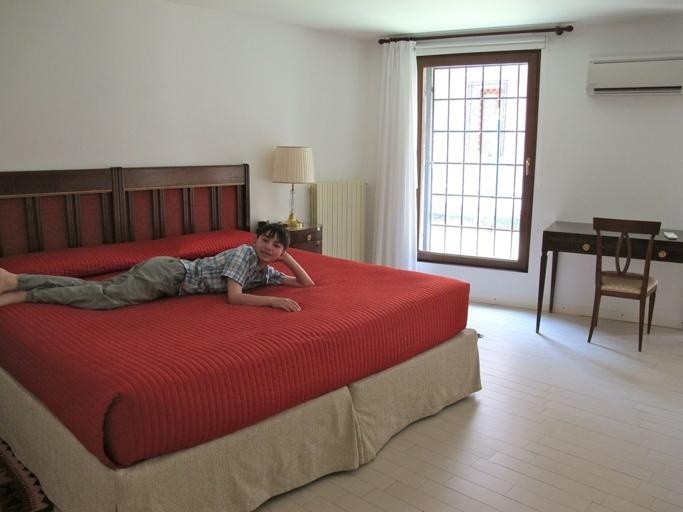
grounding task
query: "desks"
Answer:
[536,220,682,334]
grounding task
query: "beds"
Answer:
[0,163,481,512]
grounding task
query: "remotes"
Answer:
[663,231,678,240]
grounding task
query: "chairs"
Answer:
[587,216,661,352]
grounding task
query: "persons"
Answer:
[0,223,315,312]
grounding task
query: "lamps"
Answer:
[271,145,315,229]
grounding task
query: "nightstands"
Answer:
[289,224,322,255]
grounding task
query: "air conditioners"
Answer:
[586,53,683,95]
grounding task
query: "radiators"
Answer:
[310,179,366,262]
